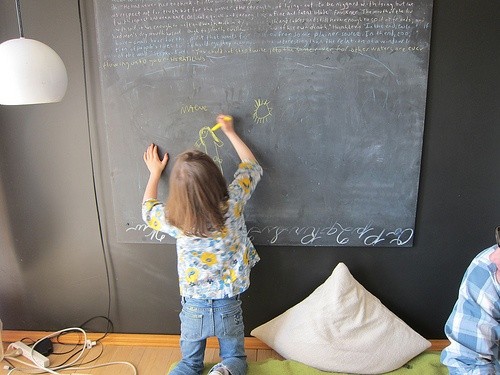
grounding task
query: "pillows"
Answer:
[250,262,433,375]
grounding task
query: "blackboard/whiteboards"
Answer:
[94,0,434,248]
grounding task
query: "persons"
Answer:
[440,224,500,375]
[141,115,263,375]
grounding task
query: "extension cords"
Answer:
[11,342,50,368]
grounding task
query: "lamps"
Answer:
[0,0,68,106]
